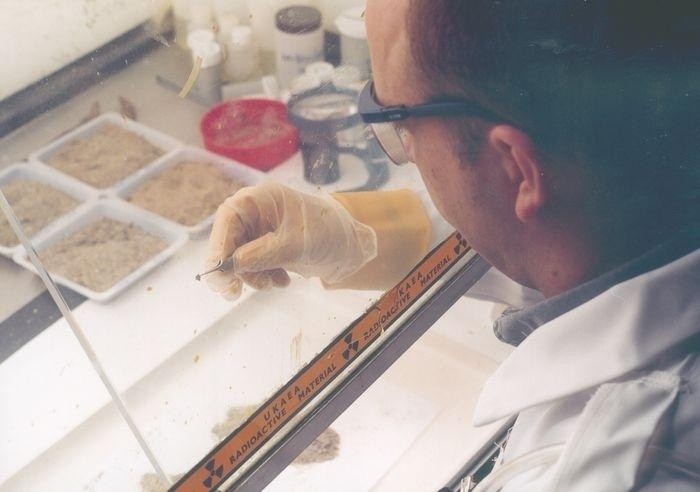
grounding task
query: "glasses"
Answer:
[358,78,529,166]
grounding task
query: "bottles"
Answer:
[173,2,261,91]
[275,4,325,90]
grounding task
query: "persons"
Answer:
[213,1,699,490]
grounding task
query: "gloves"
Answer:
[205,182,431,302]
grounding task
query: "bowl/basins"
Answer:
[200,97,300,171]
